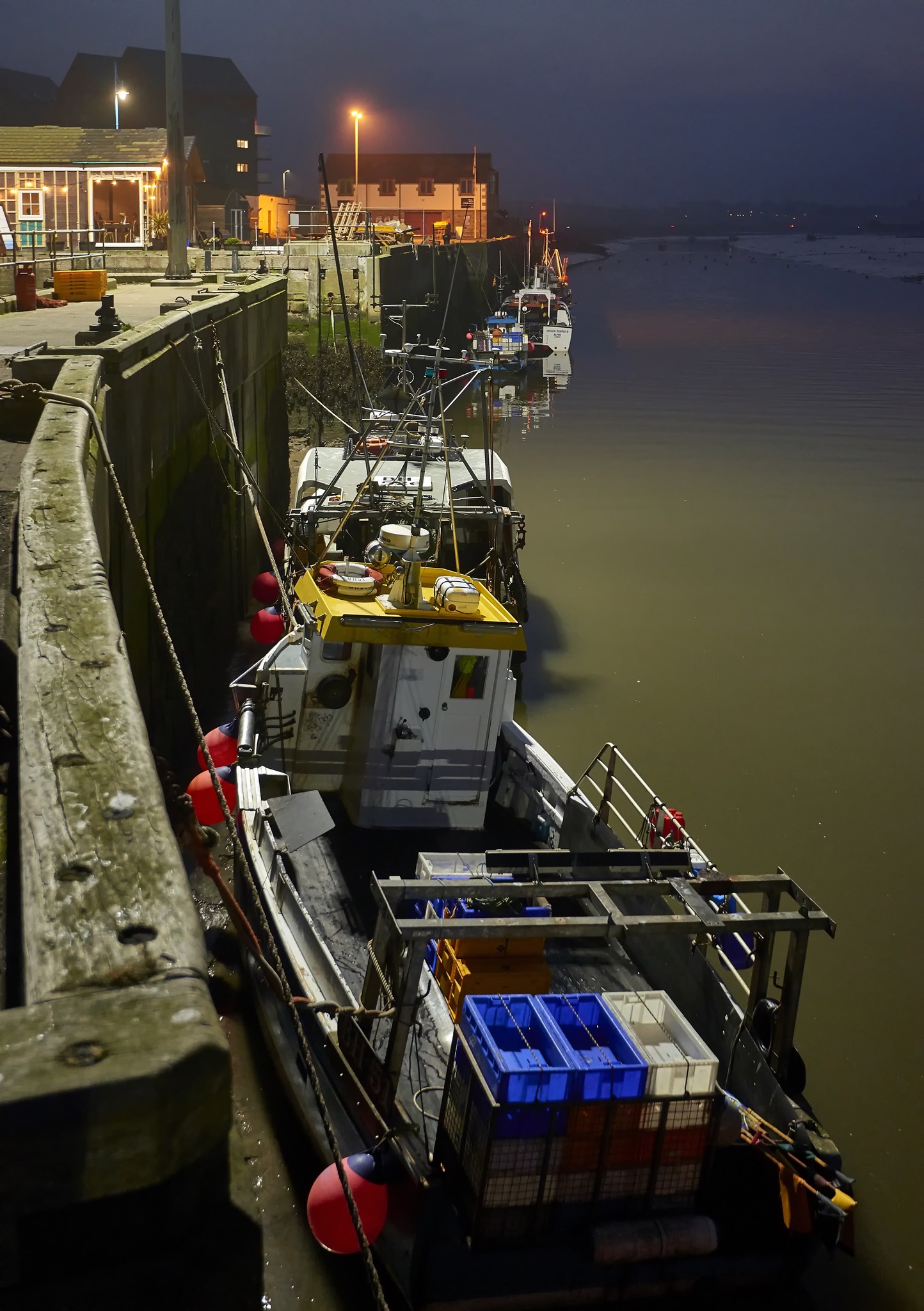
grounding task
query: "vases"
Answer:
[78,241,97,252]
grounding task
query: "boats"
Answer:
[466,198,573,395]
[190,302,855,1250]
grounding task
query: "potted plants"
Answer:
[223,237,254,252]
[201,236,222,251]
[146,207,170,251]
[44,236,66,252]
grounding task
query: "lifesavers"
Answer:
[318,562,383,596]
[357,437,392,454]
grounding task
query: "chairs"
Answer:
[94,219,138,243]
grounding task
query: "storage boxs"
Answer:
[433,220,450,241]
[405,847,724,1210]
[476,329,530,353]
[50,268,109,302]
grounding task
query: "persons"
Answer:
[448,654,481,699]
[539,312,553,332]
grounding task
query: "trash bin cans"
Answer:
[15,262,37,311]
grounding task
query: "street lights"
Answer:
[539,211,546,232]
[351,109,362,206]
[114,64,129,130]
[283,170,291,198]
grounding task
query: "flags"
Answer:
[528,221,532,236]
[492,276,496,287]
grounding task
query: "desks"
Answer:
[104,222,133,243]
